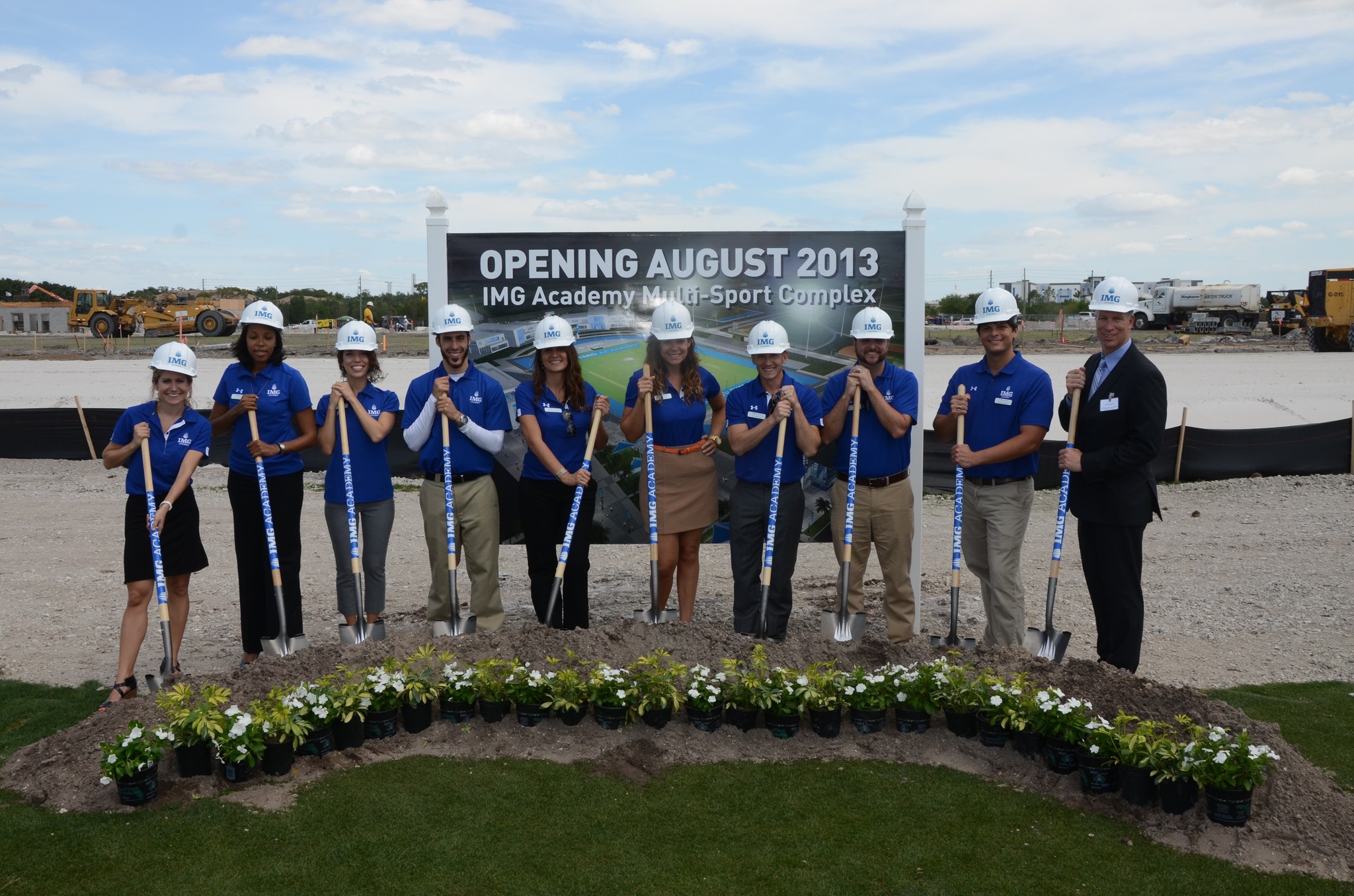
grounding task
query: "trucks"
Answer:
[381,315,413,330]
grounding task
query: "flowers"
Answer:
[99,645,1283,792]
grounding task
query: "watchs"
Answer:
[708,435,721,446]
[159,501,172,511]
[457,415,469,428]
[276,443,285,455]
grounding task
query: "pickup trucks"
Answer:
[1065,312,1096,322]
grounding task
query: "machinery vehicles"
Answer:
[66,288,245,337]
[1263,267,1354,353]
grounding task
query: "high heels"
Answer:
[239,651,260,667]
[159,655,182,678]
[99,674,138,710]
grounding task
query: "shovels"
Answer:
[929,382,979,651]
[1019,384,1080,661]
[338,393,386,646]
[818,382,869,644]
[430,389,477,640]
[138,424,188,691]
[633,363,679,626]
[739,397,785,646]
[248,407,309,660]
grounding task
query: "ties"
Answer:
[1088,358,1105,402]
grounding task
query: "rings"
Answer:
[952,456,954,460]
[859,368,861,371]
[957,405,962,410]
[605,405,606,408]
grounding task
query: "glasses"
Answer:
[562,410,577,440]
[769,393,780,415]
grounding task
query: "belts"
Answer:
[836,467,909,488]
[738,479,801,488]
[964,474,1031,486]
[424,470,488,483]
[653,439,706,454]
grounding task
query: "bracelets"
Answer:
[555,468,568,481]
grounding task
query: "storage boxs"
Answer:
[1178,335,1190,347]
[1183,321,1189,327]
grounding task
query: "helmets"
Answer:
[533,315,576,349]
[396,323,399,325]
[849,307,895,339]
[431,303,474,335]
[1088,276,1140,313]
[147,341,198,379]
[335,320,379,352]
[650,301,694,340]
[404,316,406,318]
[238,300,285,334]
[747,320,791,354]
[367,301,374,306]
[974,288,1021,324]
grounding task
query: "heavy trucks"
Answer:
[1129,280,1262,336]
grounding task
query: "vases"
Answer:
[113,696,1252,824]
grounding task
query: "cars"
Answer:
[953,318,976,325]
[285,318,337,329]
[925,318,928,324]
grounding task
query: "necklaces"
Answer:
[247,375,269,395]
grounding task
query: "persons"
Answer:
[620,298,727,622]
[364,301,380,342]
[399,304,514,630]
[314,317,400,623]
[390,315,407,332]
[1058,277,1168,672]
[98,341,213,713]
[514,316,612,630]
[821,306,919,645]
[726,320,822,641]
[933,286,1054,651]
[206,300,316,669]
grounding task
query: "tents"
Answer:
[336,316,356,328]
